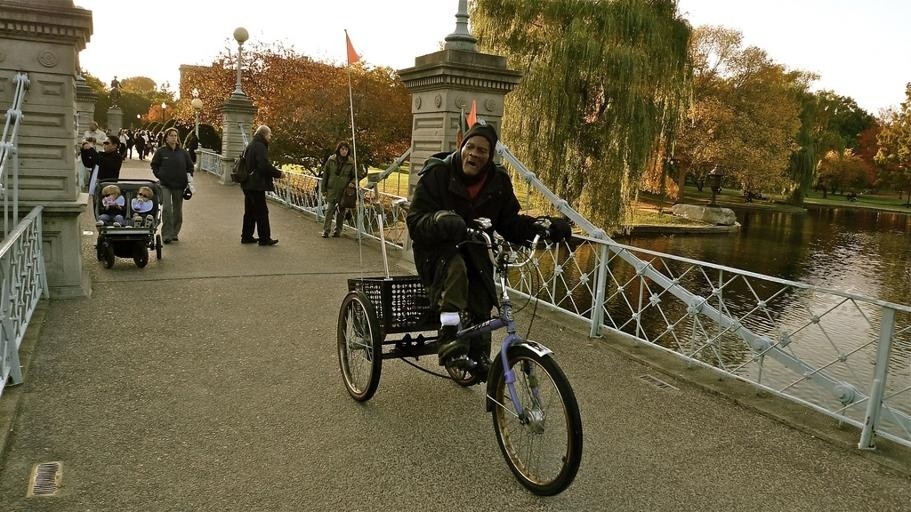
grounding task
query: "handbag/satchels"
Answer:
[183,187,191,200]
[341,186,355,208]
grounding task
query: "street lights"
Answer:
[137,113,142,128]
[161,102,167,126]
[191,87,204,142]
[230,27,252,95]
[708,162,724,208]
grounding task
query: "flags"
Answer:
[347,34,363,67]
[469,101,476,128]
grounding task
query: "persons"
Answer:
[96,185,127,227]
[102,127,164,160]
[237,126,285,247]
[132,186,156,228]
[80,135,127,221]
[408,120,572,373]
[83,120,109,154]
[108,75,125,109]
[320,140,362,240]
[150,125,195,246]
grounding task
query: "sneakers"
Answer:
[322,231,328,237]
[333,230,340,237]
[145,215,153,227]
[134,216,142,228]
[113,221,120,227]
[95,220,104,226]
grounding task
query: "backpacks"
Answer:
[231,140,256,183]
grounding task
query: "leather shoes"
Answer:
[163,239,170,244]
[260,237,278,246]
[242,236,259,243]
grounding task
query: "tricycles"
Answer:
[331,216,586,497]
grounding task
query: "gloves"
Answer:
[434,210,466,243]
[532,217,571,243]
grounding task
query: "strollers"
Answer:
[91,177,165,271]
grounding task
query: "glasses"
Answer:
[103,141,111,145]
[102,193,112,198]
[139,192,149,198]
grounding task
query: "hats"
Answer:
[459,122,498,162]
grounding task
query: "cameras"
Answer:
[86,137,97,144]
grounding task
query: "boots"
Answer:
[436,325,469,366]
[472,343,493,380]
